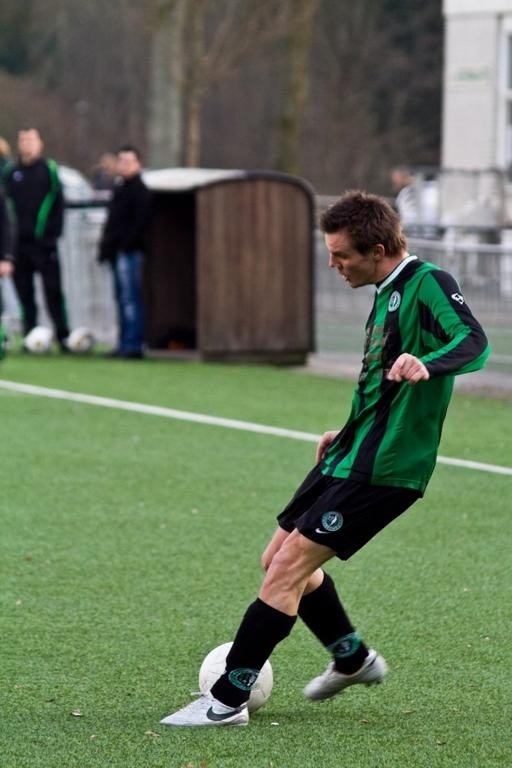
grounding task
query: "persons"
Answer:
[1,127,75,352]
[1,139,18,359]
[98,144,157,356]
[389,165,421,223]
[159,190,491,726]
[92,152,116,187]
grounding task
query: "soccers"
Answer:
[23,326,52,355]
[199,643,274,713]
[69,328,94,353]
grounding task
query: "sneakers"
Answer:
[159,689,249,726]
[303,648,387,700]
